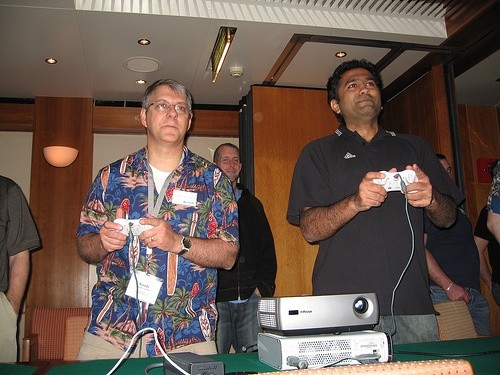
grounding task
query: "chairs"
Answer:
[64,316,90,361]
[433,300,493,341]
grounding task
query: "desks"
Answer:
[0,337,500,375]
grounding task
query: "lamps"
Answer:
[43,146,78,168]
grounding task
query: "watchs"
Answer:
[178,236,193,256]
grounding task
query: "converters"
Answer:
[164,352,225,375]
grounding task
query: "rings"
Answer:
[150,236,154,241]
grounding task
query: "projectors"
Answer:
[257,292,380,336]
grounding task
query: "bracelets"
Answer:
[445,281,454,291]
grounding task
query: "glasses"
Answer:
[146,102,193,115]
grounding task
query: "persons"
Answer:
[287,58,500,342]
[76,80,239,361]
[213,143,277,354]
[0,176,42,363]
